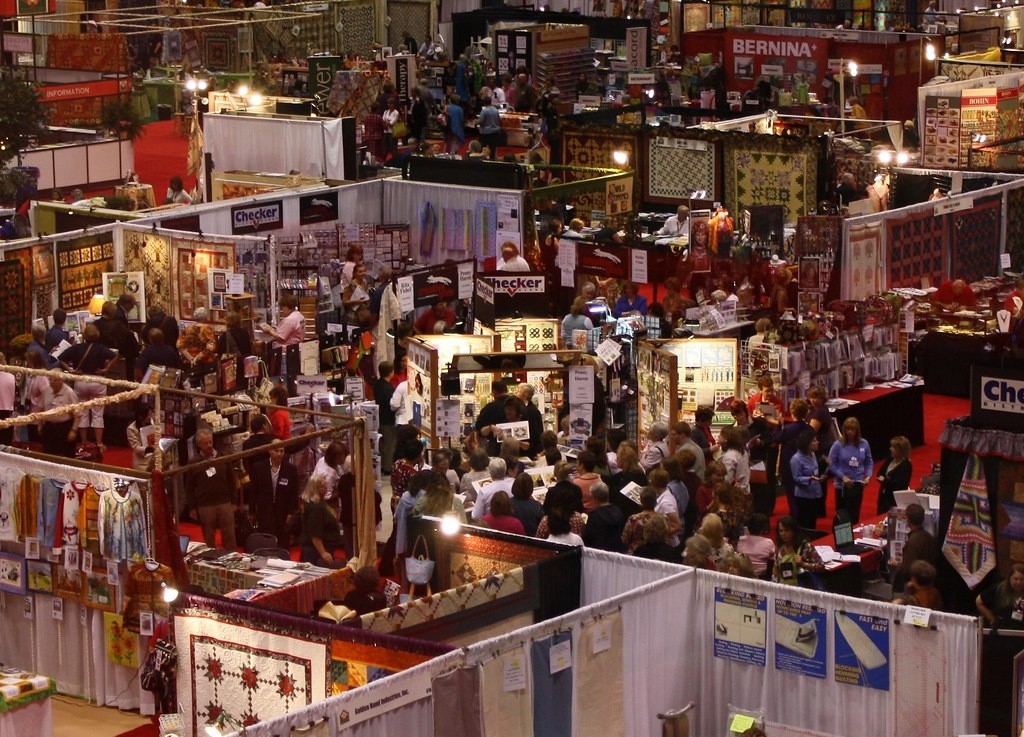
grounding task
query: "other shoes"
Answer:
[98,443,107,452]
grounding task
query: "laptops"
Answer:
[180,534,190,557]
[833,521,873,556]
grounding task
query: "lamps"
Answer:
[442,510,461,537]
[970,130,986,143]
[159,436,180,467]
[142,222,158,247]
[613,151,632,170]
[160,581,179,643]
[768,109,777,121]
[641,89,655,102]
[64,208,74,215]
[185,79,209,105]
[236,85,262,112]
[997,36,1012,45]
[189,229,204,258]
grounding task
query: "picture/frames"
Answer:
[204,36,230,70]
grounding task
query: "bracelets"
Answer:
[803,562,809,569]
[71,429,78,433]
[489,424,492,431]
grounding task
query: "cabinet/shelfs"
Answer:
[225,293,259,353]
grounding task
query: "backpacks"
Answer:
[779,540,807,586]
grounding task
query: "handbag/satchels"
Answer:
[141,617,179,691]
[643,446,665,475]
[250,359,274,404]
[438,105,448,126]
[405,533,434,584]
[775,470,782,485]
[391,114,407,138]
[437,105,450,135]
[748,448,768,484]
[831,417,842,440]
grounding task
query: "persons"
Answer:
[0,0,1024,721]
[764,254,787,285]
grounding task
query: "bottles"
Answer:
[859,524,873,538]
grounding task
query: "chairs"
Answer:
[246,533,278,558]
